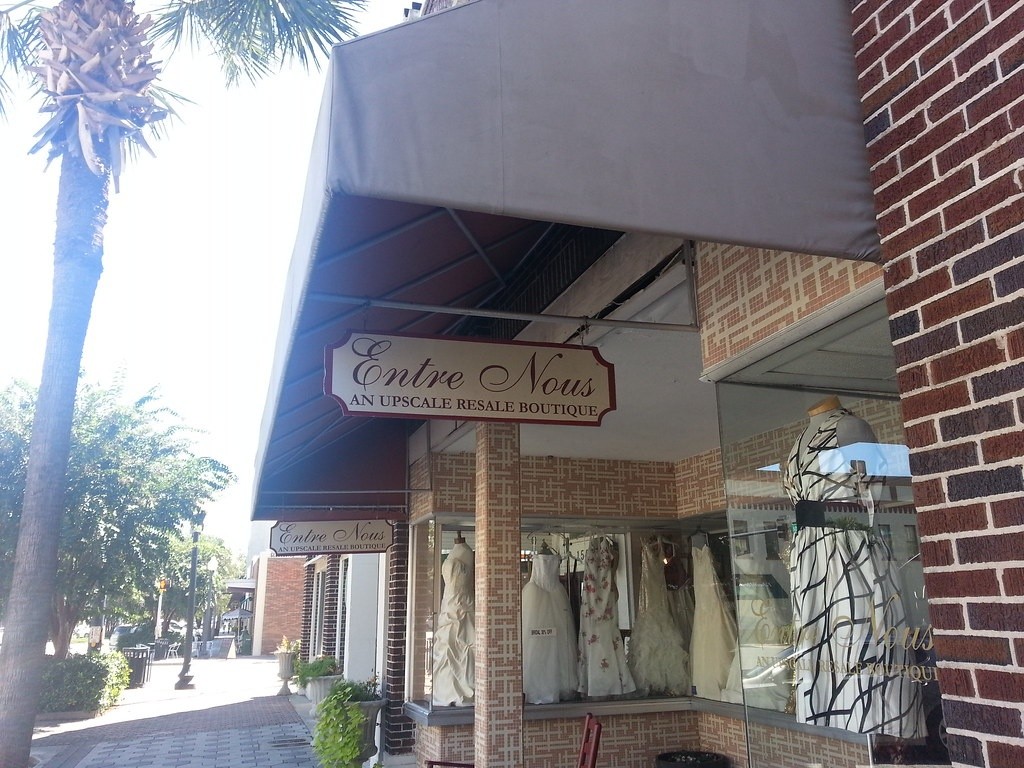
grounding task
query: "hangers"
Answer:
[650,534,680,550]
[526,527,567,543]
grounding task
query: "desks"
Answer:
[148,643,155,659]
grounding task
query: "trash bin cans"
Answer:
[123,647,150,688]
[154,638,170,661]
[656,751,728,768]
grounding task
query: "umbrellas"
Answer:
[223,607,253,647]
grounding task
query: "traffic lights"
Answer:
[166,580,172,588]
[159,578,166,589]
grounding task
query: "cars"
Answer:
[161,619,184,635]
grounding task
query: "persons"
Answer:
[195,630,201,637]
[222,623,232,634]
[433,530,475,707]
[783,397,927,738]
[522,541,577,703]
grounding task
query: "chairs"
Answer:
[135,632,244,659]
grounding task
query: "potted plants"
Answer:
[313,668,386,768]
[299,655,342,745]
[275,635,301,694]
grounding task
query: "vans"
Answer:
[109,624,180,653]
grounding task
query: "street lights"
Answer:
[175,509,205,690]
[204,555,218,641]
